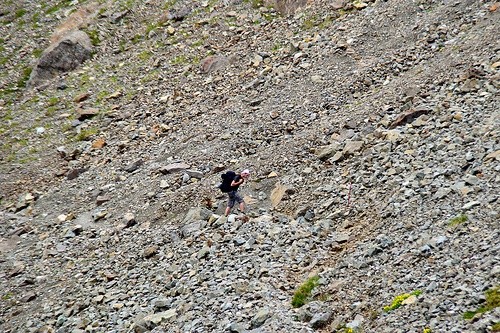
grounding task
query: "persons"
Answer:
[224,168,250,217]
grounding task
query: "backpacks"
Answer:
[218,170,236,192]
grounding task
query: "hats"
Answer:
[242,169,250,175]
